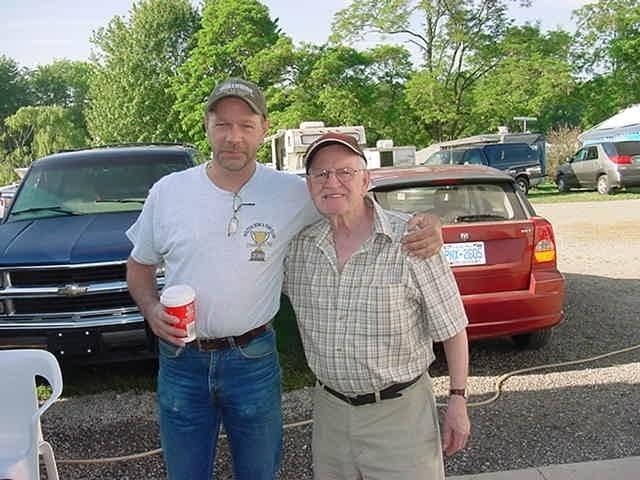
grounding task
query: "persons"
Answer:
[282,133,473,480]
[125,78,444,480]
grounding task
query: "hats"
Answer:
[302,131,367,167]
[207,78,267,115]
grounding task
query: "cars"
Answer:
[552,138,640,194]
[366,162,567,351]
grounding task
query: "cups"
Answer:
[157,284,200,343]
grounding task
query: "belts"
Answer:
[317,372,422,407]
[190,323,268,352]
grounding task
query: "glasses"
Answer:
[308,168,365,184]
[226,193,256,237]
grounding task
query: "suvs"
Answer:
[418,142,548,196]
[0,143,205,374]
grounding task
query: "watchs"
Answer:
[449,387,469,399]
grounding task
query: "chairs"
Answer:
[0,349,63,480]
[424,188,471,223]
